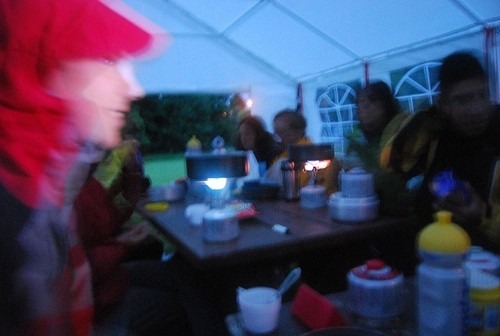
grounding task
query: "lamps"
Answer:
[184,153,251,244]
[288,142,335,210]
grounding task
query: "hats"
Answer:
[437,52,488,92]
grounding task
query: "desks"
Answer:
[133,184,419,272]
[223,277,417,336]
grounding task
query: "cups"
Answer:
[281,162,299,201]
[237,287,281,332]
[202,211,239,242]
[415,212,469,336]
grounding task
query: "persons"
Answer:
[231,52,500,258]
[0,0,188,336]
